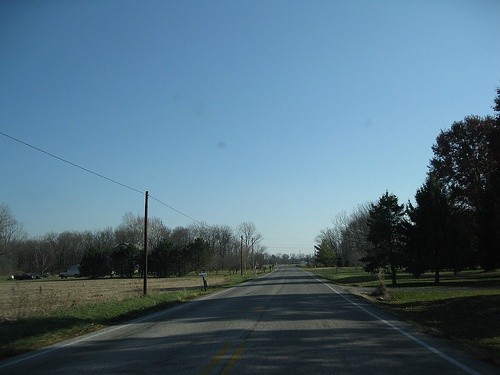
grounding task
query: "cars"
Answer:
[9,273,41,280]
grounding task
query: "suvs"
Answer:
[59,267,80,279]
